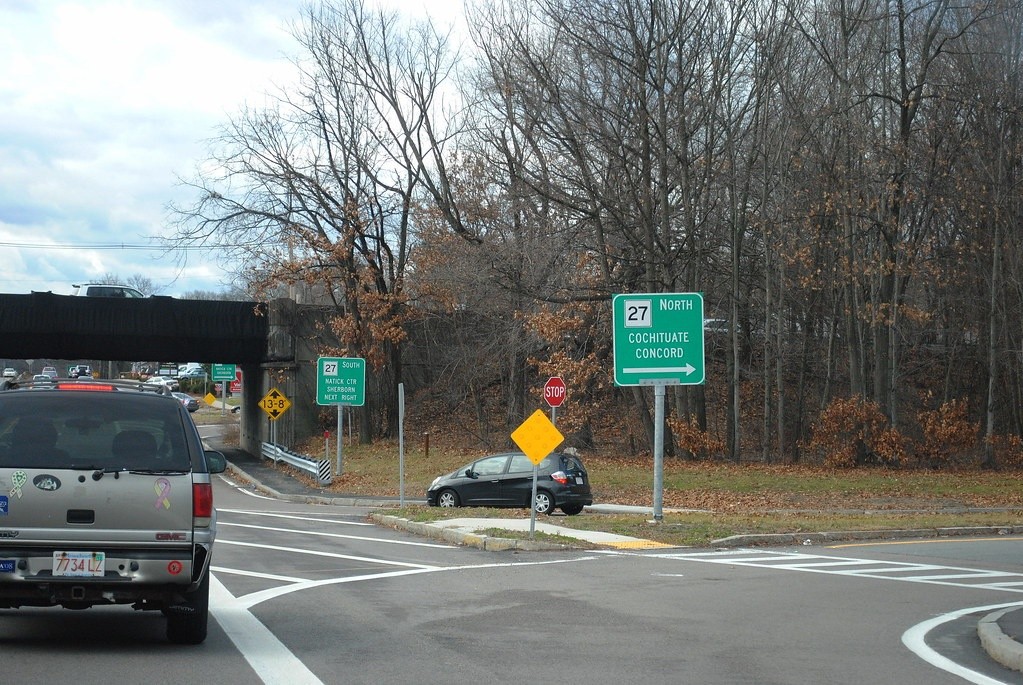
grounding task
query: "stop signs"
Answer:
[544,376,567,408]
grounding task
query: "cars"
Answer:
[1,361,213,421]
[74,281,153,299]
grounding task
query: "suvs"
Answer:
[426,451,594,517]
[1,378,227,647]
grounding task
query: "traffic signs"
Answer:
[613,293,707,387]
[315,356,366,407]
[211,363,236,381]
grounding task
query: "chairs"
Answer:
[0,417,72,469]
[111,429,158,471]
